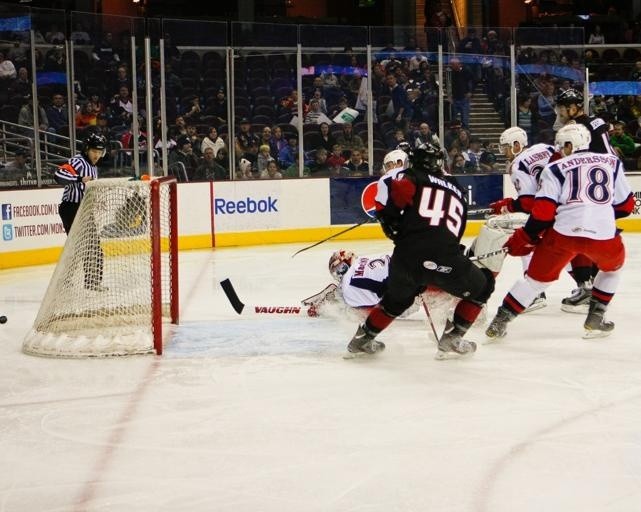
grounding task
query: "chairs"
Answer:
[520,47,640,81]
[0,31,100,126]
[298,53,398,162]
[162,50,299,137]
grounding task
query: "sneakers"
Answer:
[584,313,615,334]
[485,318,508,339]
[437,318,477,356]
[85,282,109,293]
[347,323,386,356]
[526,292,545,299]
[561,287,591,306]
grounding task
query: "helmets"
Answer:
[85,132,106,157]
[555,123,591,153]
[499,126,528,154]
[554,88,585,114]
[328,249,357,281]
[407,142,445,178]
[382,149,409,172]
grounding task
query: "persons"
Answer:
[329,212,530,314]
[611,94,639,124]
[365,149,411,217]
[348,145,493,359]
[555,89,610,315]
[491,126,558,314]
[54,135,110,292]
[486,125,633,339]
[556,32,638,88]
[501,37,554,125]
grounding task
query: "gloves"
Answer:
[502,226,534,256]
[489,197,514,215]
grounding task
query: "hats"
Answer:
[239,158,252,177]
